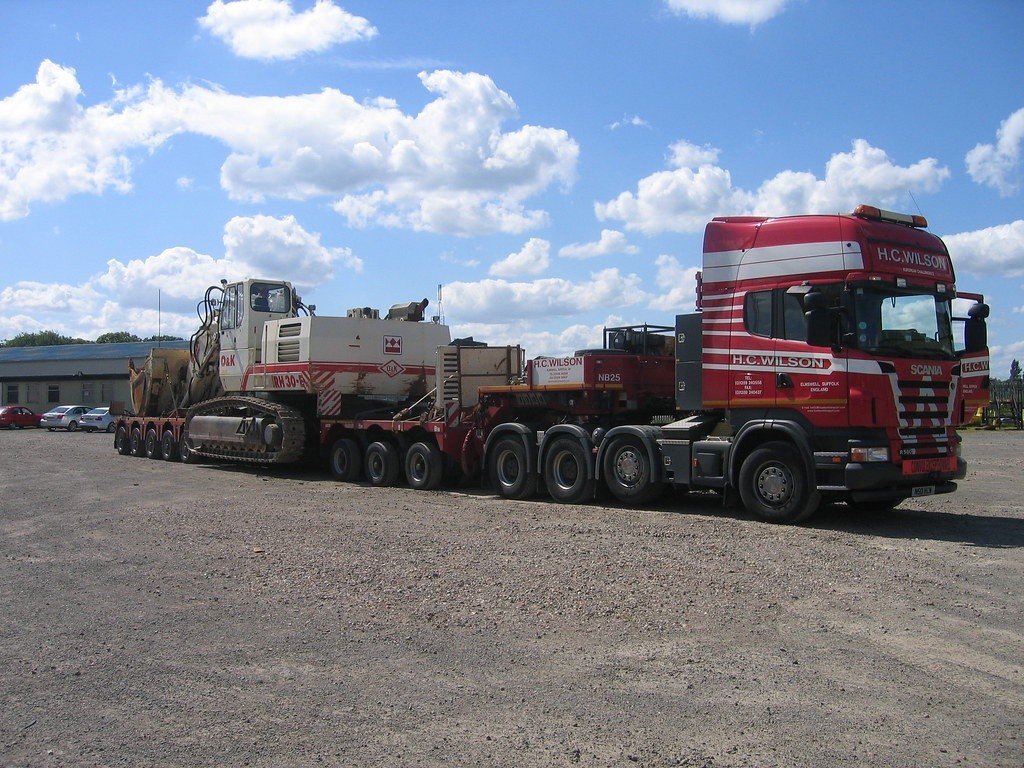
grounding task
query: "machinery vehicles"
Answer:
[127,278,453,462]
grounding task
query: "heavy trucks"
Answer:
[114,202,990,525]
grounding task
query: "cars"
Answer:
[79,407,125,433]
[40,405,95,431]
[0,405,42,430]
